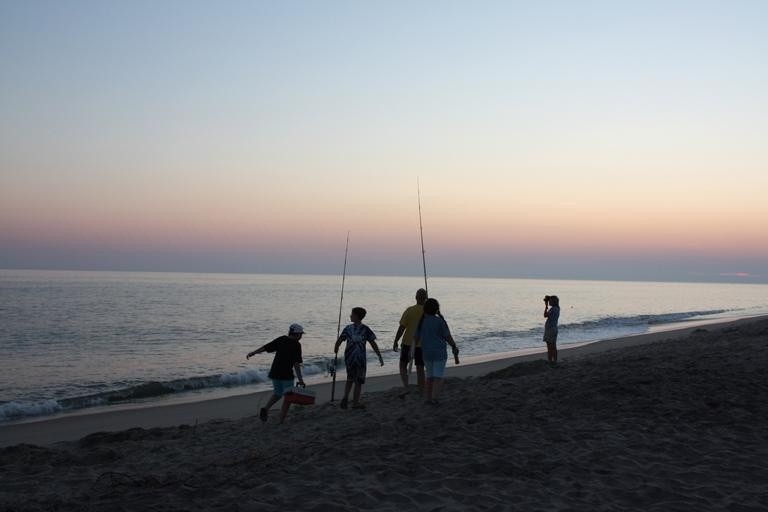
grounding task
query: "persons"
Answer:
[391,288,428,399]
[333,306,385,411]
[415,297,459,408]
[543,294,561,363]
[244,322,307,424]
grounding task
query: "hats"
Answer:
[289,323,305,334]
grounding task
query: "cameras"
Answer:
[544,296,550,301]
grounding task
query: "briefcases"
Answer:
[282,381,316,406]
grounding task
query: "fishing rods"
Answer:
[331,230,350,403]
[417,177,428,300]
